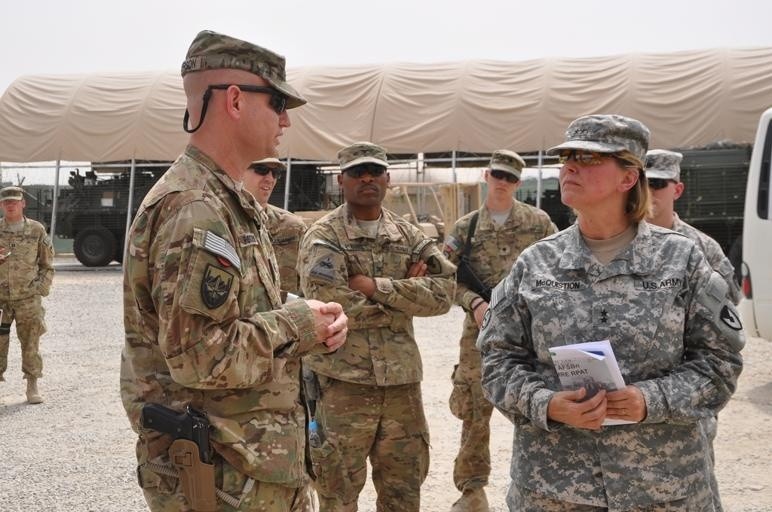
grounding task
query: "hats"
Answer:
[644,148,683,184]
[1,185,26,203]
[489,149,526,179]
[336,142,390,171]
[248,148,287,172]
[546,114,650,167]
[181,30,308,112]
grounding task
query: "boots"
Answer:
[450,489,491,512]
[26,377,44,405]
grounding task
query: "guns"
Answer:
[140,402,212,464]
[457,256,491,304]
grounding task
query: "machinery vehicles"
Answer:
[385,181,448,249]
[38,161,332,269]
[523,141,749,286]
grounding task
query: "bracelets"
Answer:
[472,300,486,310]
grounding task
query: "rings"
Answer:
[622,409,626,415]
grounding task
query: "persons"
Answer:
[240,157,306,303]
[118,30,347,512]
[646,149,742,306]
[293,141,456,512]
[475,114,746,512]
[0,187,55,403]
[443,148,559,512]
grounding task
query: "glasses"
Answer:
[251,164,281,179]
[557,149,609,167]
[490,169,519,184]
[347,165,387,179]
[647,177,668,191]
[209,81,290,114]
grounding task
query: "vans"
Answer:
[736,103,772,347]
